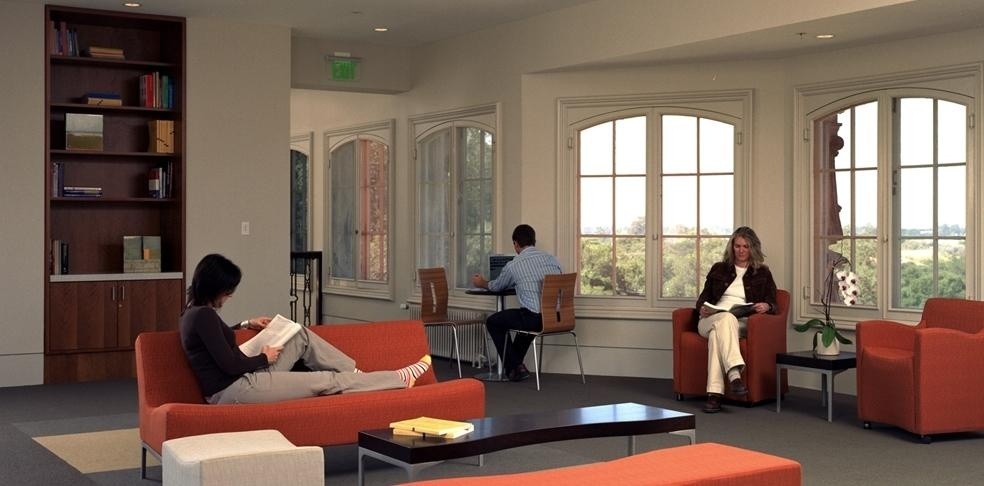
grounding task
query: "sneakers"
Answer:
[508,365,529,381]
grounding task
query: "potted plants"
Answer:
[790,244,852,355]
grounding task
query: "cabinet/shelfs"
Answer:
[43,279,187,355]
[42,4,184,280]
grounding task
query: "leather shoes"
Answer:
[704,394,720,412]
[730,378,747,395]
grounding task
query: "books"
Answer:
[64,112,103,152]
[389,415,474,436]
[237,313,302,357]
[139,71,175,199]
[703,297,755,319]
[122,235,160,273]
[82,97,121,107]
[52,160,102,199]
[49,18,127,59]
[52,239,69,276]
[392,426,473,439]
[77,91,121,100]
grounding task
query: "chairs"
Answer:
[497,273,587,390]
[418,268,492,382]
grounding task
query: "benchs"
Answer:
[399,440,801,485]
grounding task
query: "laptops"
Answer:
[490,256,515,282]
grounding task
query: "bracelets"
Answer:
[240,319,250,330]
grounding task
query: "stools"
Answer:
[157,429,324,485]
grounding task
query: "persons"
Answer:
[470,223,565,382]
[692,226,779,416]
[179,252,433,405]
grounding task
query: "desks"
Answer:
[465,286,516,373]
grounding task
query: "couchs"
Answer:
[854,297,979,444]
[671,288,790,409]
[132,321,488,479]
